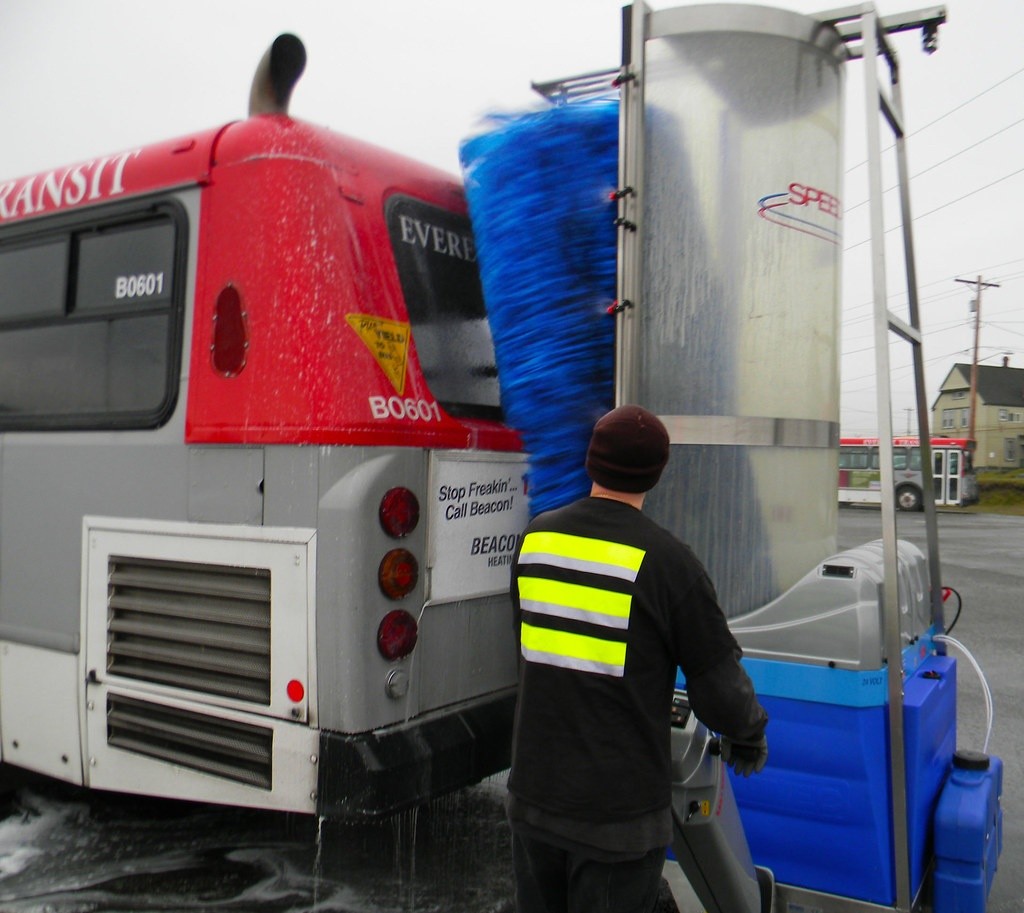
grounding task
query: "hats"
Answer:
[586,404,669,493]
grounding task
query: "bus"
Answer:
[837,437,981,513]
[0,29,518,832]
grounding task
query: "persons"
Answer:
[505,405,768,913]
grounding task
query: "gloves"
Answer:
[721,734,768,777]
